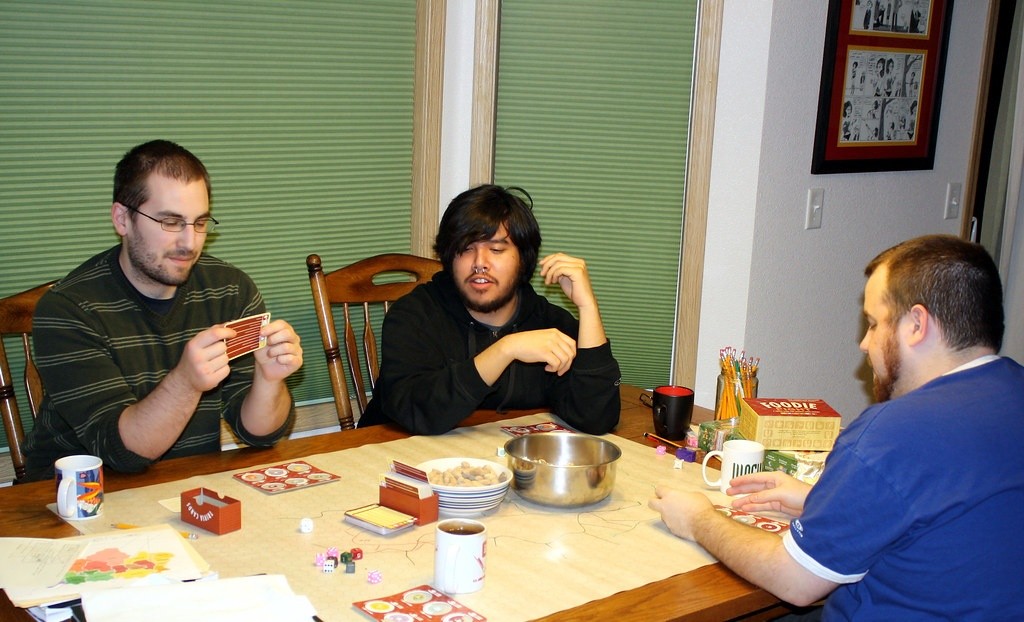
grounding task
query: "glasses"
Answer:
[125,206,219,233]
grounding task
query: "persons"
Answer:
[648,234,1024,622]
[357,183,622,435]
[12,140,304,486]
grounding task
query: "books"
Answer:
[0,522,217,622]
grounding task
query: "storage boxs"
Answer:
[698,418,829,486]
[379,486,440,526]
[739,398,842,452]
[181,488,242,535]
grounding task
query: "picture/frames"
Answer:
[811,0,955,175]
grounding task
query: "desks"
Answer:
[0,383,831,622]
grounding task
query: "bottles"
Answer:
[714,371,759,422]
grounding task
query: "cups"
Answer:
[434,518,487,593]
[701,440,765,497]
[54,455,105,521]
[651,386,694,440]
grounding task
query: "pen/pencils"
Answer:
[715,346,760,421]
[644,432,683,449]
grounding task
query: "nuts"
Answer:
[429,461,507,487]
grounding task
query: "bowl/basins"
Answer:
[415,458,513,516]
[504,434,622,508]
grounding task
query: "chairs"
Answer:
[305,252,443,432]
[0,279,61,481]
